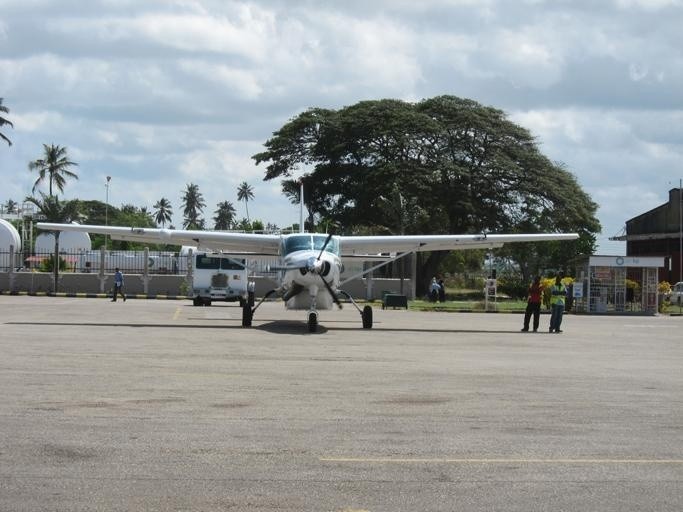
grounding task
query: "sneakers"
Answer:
[520,328,538,332]
[548,328,564,333]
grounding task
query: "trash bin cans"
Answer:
[596,297,607,312]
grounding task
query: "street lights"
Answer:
[105,176,111,250]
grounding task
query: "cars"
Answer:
[670,282,683,307]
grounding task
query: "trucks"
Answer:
[193,254,255,307]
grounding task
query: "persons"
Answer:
[437,272,446,302]
[520,274,544,332]
[428,276,440,301]
[548,274,567,334]
[110,267,126,302]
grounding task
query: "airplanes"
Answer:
[35,183,580,331]
